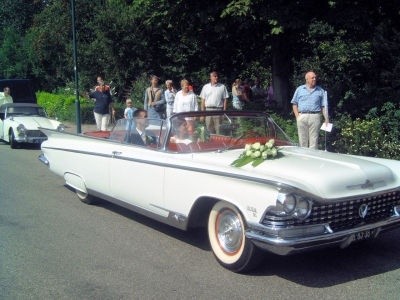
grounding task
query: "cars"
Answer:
[0,103,64,149]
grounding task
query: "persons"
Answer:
[199,71,229,135]
[88,76,114,131]
[290,71,329,149]
[170,118,192,145]
[125,109,156,145]
[143,74,198,129]
[0,87,14,113]
[124,99,138,130]
[231,77,273,110]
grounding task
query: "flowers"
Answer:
[231,137,278,168]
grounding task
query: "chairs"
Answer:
[198,138,232,148]
[150,143,188,152]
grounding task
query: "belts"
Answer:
[298,111,320,114]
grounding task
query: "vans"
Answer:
[1,79,37,103]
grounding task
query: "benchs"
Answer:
[86,129,172,146]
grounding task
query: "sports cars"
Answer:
[38,110,400,273]
